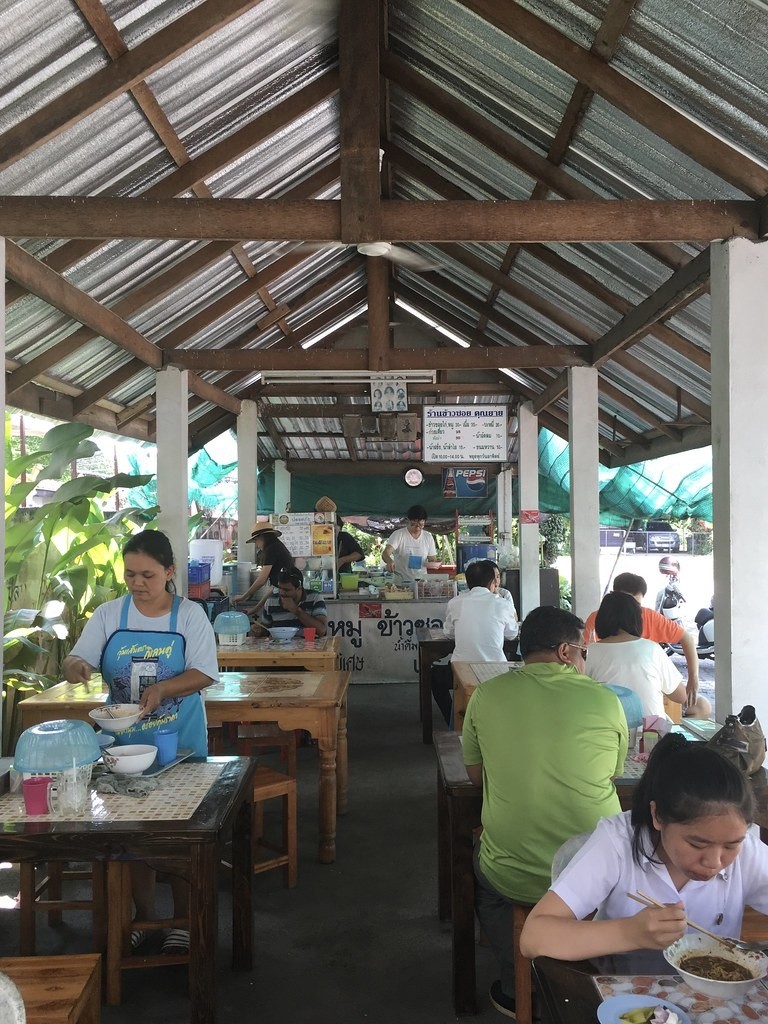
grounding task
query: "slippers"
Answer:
[160,928,190,955]
[130,928,155,949]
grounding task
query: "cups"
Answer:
[153,729,178,766]
[304,628,316,641]
[21,777,52,815]
[191,560,199,575]
[47,774,88,818]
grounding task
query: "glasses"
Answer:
[551,642,587,662]
[280,567,301,581]
[409,520,424,529]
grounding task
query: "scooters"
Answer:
[654,573,716,661]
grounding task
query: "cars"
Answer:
[627,521,680,552]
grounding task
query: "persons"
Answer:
[63,530,218,955]
[518,733,768,959]
[583,572,711,718]
[383,505,442,583]
[462,604,628,1019]
[483,560,521,662]
[432,561,519,726]
[250,567,326,670]
[373,386,405,411]
[337,515,365,573]
[234,521,294,615]
[585,591,686,724]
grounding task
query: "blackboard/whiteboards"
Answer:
[420,404,508,463]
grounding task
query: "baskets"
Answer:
[214,611,250,645]
[14,717,103,774]
[19,743,93,798]
[604,683,643,729]
[628,728,637,749]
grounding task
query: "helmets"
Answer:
[659,556,680,575]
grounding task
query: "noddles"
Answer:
[682,955,752,982]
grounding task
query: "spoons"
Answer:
[725,938,768,951]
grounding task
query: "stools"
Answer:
[18,858,105,957]
[249,765,298,889]
[104,858,196,1009]
[236,724,298,783]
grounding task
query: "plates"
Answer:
[597,995,691,1024]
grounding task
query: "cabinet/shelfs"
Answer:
[456,510,494,545]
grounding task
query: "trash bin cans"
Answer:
[686,537,696,551]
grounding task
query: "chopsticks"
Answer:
[627,890,736,948]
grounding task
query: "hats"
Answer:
[245,522,282,543]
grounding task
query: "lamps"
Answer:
[281,147,441,274]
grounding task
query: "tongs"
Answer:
[394,571,400,576]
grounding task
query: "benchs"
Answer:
[512,905,768,1024]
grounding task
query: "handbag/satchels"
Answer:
[710,704,768,775]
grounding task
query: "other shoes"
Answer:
[304,729,317,745]
[488,980,538,1020]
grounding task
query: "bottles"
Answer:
[588,628,597,643]
[442,468,457,497]
[10,765,24,794]
[251,563,270,600]
[507,554,520,568]
[644,732,658,753]
[303,576,332,591]
[223,565,237,596]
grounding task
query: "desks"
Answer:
[451,660,528,727]
[414,622,528,743]
[210,628,344,674]
[12,670,353,865]
[0,950,103,1023]
[0,749,257,994]
[430,725,768,1019]
[533,941,768,1024]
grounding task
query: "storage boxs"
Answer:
[187,580,210,601]
[188,563,211,584]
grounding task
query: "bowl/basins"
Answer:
[268,628,299,641]
[102,745,157,775]
[663,934,768,1000]
[424,562,442,569]
[91,734,115,770]
[89,704,144,732]
[340,566,384,590]
